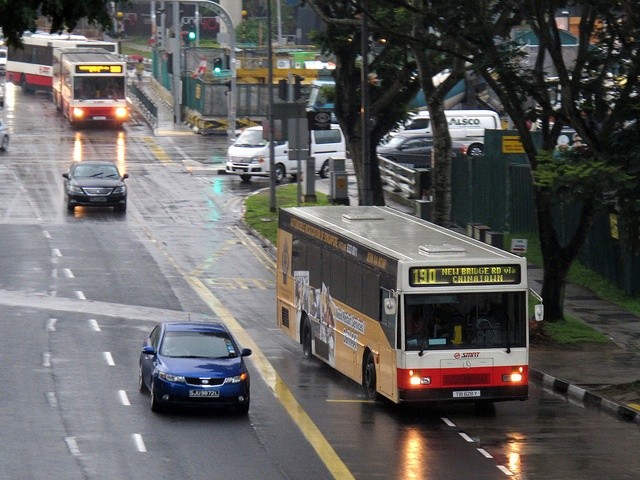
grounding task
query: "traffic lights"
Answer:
[294,73,306,100]
[182,23,196,41]
[241,11,247,42]
[212,57,222,73]
[117,12,123,57]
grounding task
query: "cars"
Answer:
[61,160,129,213]
[376,133,467,168]
[137,320,252,417]
[0,118,11,150]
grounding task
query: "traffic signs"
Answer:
[502,135,527,153]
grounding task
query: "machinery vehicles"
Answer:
[306,68,469,123]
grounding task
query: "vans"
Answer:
[383,110,500,156]
[224,123,346,183]
[0,49,6,72]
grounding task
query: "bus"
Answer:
[52,48,128,129]
[276,205,544,405]
[6,36,119,94]
